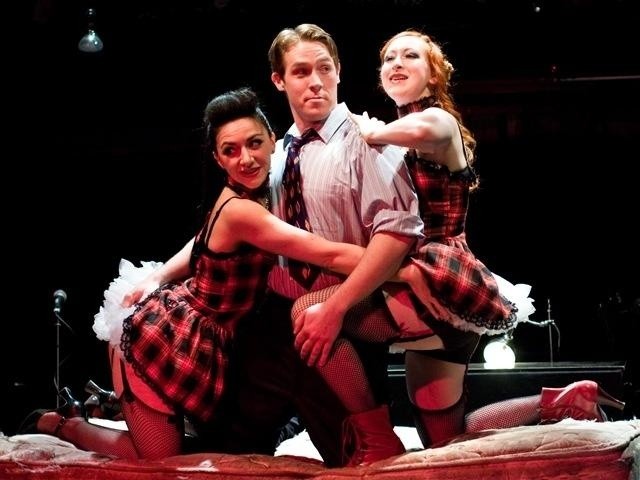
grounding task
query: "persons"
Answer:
[14,85,455,464]
[117,20,430,474]
[286,26,633,471]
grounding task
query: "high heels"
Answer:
[17,386,88,435]
[539,379,626,424]
[83,380,122,422]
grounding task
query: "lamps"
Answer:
[483,319,555,368]
[79,0,104,53]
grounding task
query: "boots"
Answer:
[341,402,408,468]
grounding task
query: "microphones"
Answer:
[53,289,68,314]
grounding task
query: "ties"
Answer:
[281,131,321,292]
